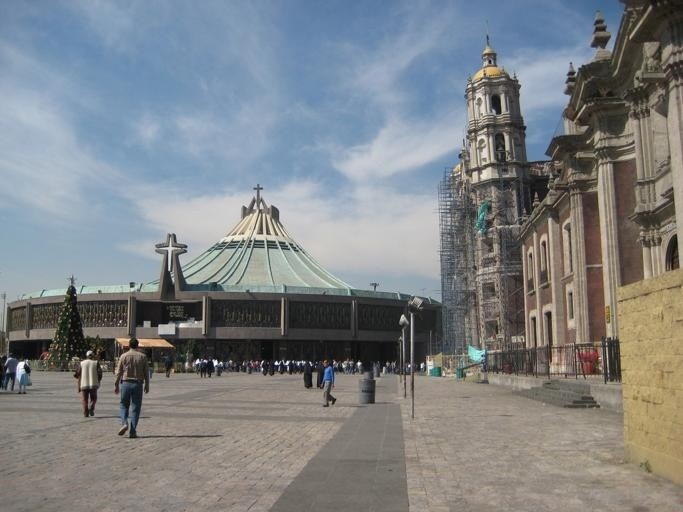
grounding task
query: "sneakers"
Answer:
[332,399,336,405]
[323,405,329,407]
[84,409,94,417]
[18,392,26,394]
[118,425,128,435]
[130,434,138,438]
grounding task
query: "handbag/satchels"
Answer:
[24,364,31,374]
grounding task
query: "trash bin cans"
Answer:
[429,367,441,377]
[358,371,376,404]
[456,368,463,378]
[373,367,380,377]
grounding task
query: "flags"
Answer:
[475,202,488,233]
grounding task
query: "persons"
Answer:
[0,353,31,394]
[115,338,149,437]
[77,351,102,417]
[148,358,154,378]
[165,358,172,377]
[185,350,425,388]
[320,359,336,407]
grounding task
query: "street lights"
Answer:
[398,293,424,421]
